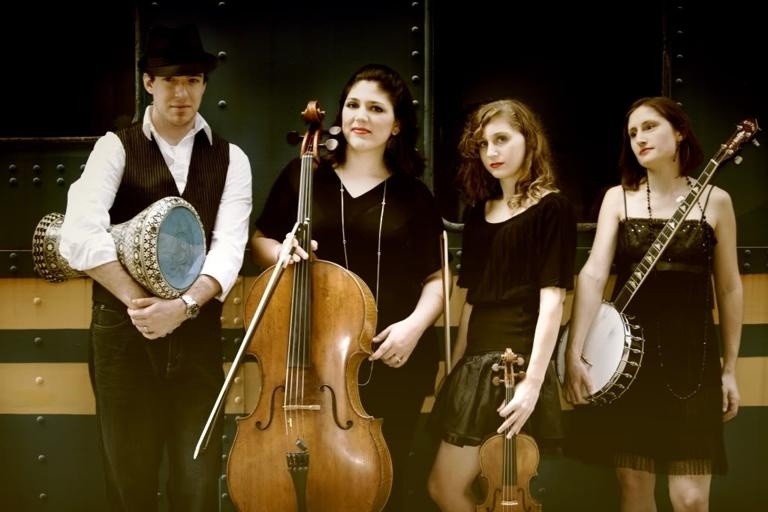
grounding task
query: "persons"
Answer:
[55,17,253,511]
[561,92,744,512]
[247,60,454,510]
[428,95,577,510]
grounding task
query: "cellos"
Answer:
[226,101,393,512]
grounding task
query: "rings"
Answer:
[394,355,401,363]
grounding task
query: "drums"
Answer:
[32,196,206,299]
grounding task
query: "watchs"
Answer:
[179,293,200,320]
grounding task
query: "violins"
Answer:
[476,348,542,512]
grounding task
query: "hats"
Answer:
[139,22,216,77]
